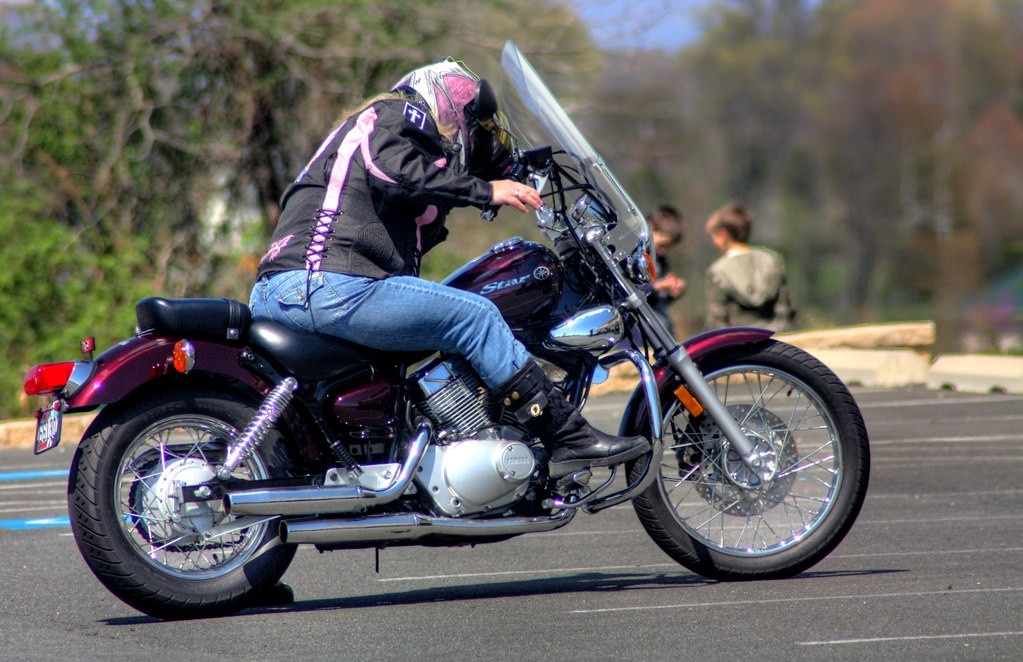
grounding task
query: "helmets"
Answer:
[389,55,515,177]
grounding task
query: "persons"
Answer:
[249,56,652,607]
[616,205,687,349]
[707,206,795,333]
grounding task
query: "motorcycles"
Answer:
[20,147,873,617]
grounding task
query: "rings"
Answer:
[516,190,519,197]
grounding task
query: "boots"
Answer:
[490,356,651,478]
